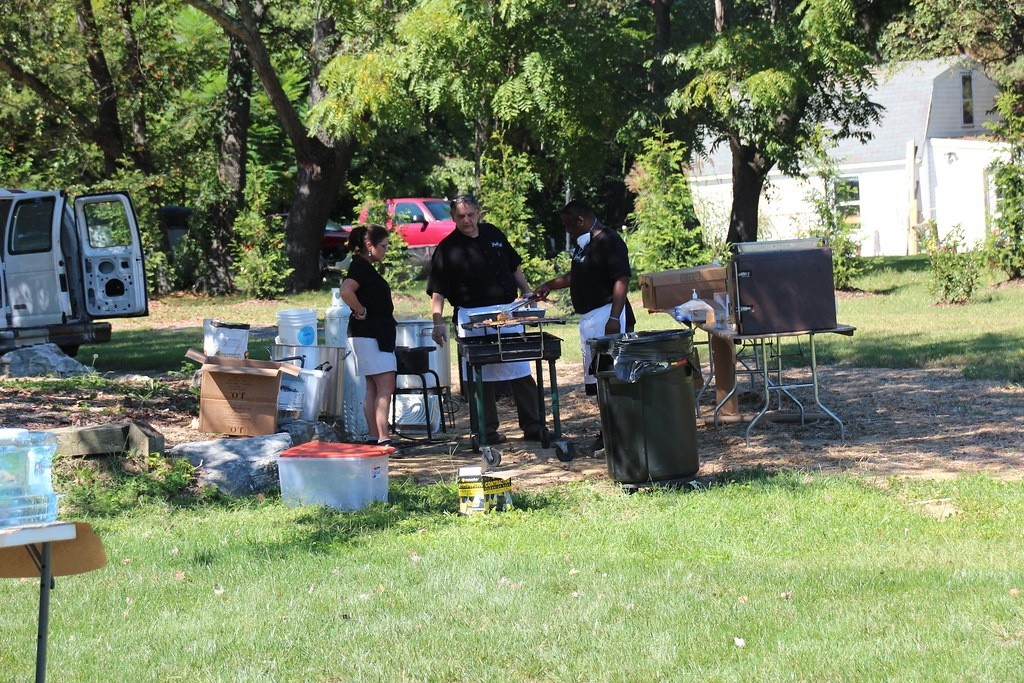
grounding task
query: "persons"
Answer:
[426,196,556,444]
[532,199,636,452]
[340,223,404,458]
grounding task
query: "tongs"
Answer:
[504,291,548,314]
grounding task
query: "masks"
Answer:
[576,233,591,250]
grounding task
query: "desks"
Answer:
[669,308,858,449]
[0,517,107,683]
[391,385,457,435]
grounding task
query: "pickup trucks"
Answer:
[320,197,456,270]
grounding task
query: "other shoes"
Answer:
[367,440,377,445]
[378,441,406,458]
[587,431,605,458]
[488,433,506,444]
[524,430,556,441]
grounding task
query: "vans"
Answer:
[0,185,149,356]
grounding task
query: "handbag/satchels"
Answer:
[203,319,250,359]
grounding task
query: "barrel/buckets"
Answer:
[267,344,352,416]
[396,321,452,389]
[203,318,249,357]
[275,309,319,347]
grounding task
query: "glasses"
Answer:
[452,196,474,203]
[373,241,386,250]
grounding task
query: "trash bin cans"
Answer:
[586,327,700,490]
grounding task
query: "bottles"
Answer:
[0,428,57,526]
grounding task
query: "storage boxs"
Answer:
[271,344,347,416]
[279,369,331,421]
[457,466,522,518]
[639,265,728,312]
[731,237,838,336]
[203,317,250,359]
[184,348,303,434]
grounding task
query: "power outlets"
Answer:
[277,442,394,512]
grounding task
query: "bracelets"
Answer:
[609,317,620,322]
[523,293,534,299]
[434,325,445,327]
[356,307,367,318]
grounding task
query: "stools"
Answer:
[392,370,445,440]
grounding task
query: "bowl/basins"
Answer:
[469,310,503,323]
[513,308,547,317]
[277,392,304,424]
